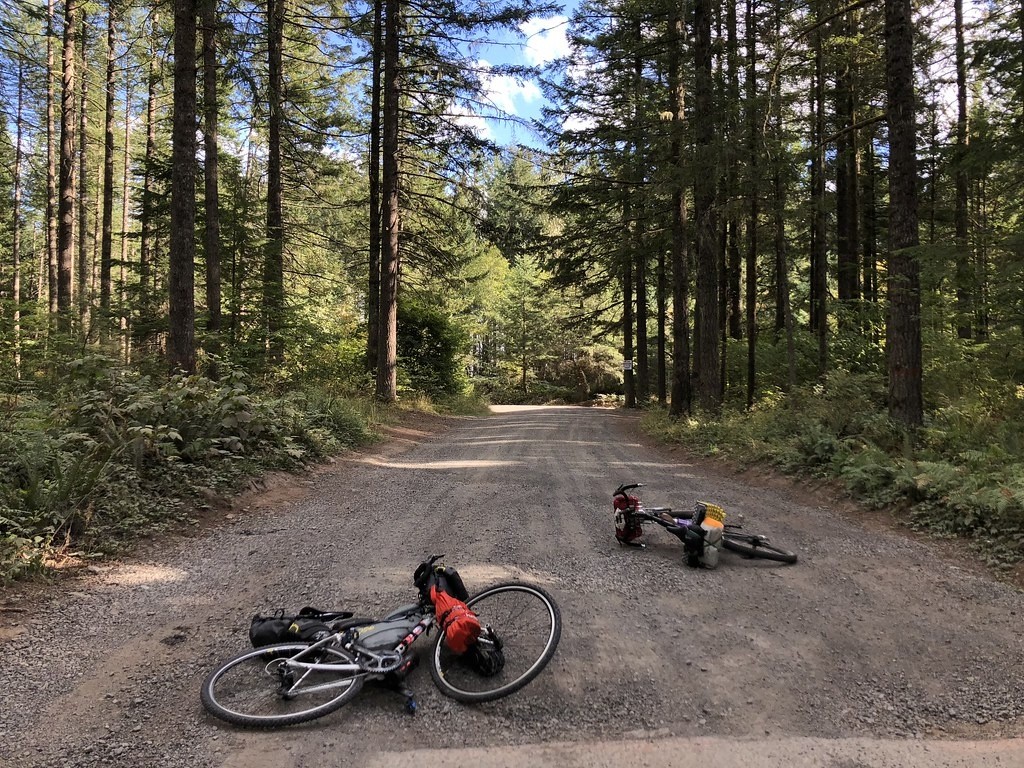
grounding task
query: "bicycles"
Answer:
[610,480,799,571]
[201,552,563,727]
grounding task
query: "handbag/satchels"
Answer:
[425,562,469,606]
[614,497,642,541]
[429,587,482,655]
[685,517,725,569]
[251,608,325,662]
